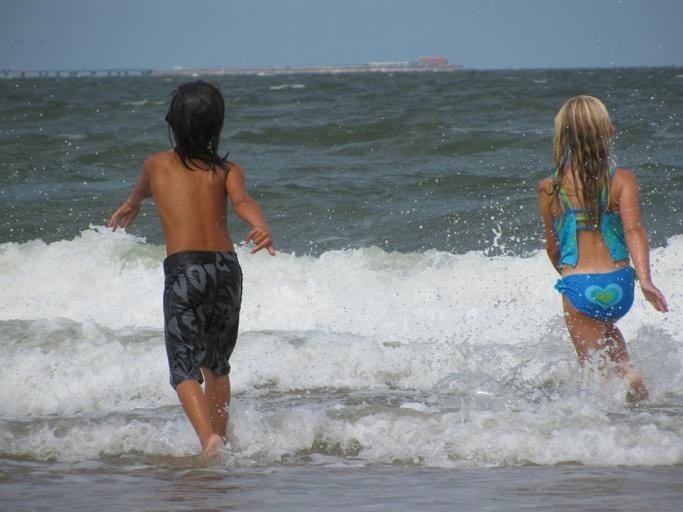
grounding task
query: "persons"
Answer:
[535,93,671,408]
[106,79,277,456]
